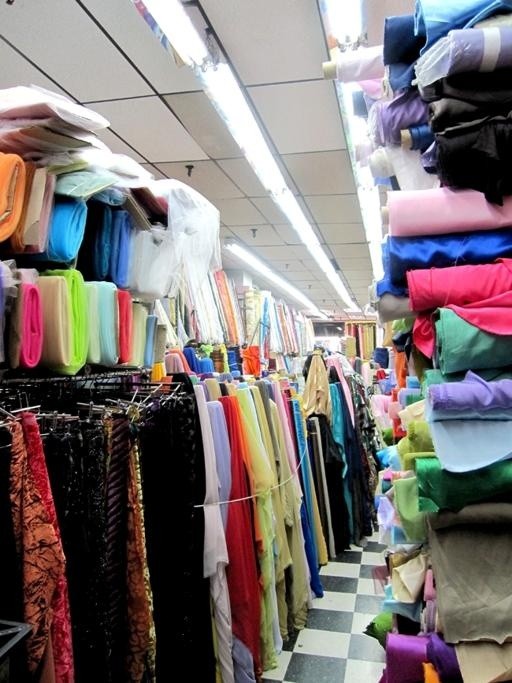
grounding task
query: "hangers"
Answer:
[1,365,190,437]
